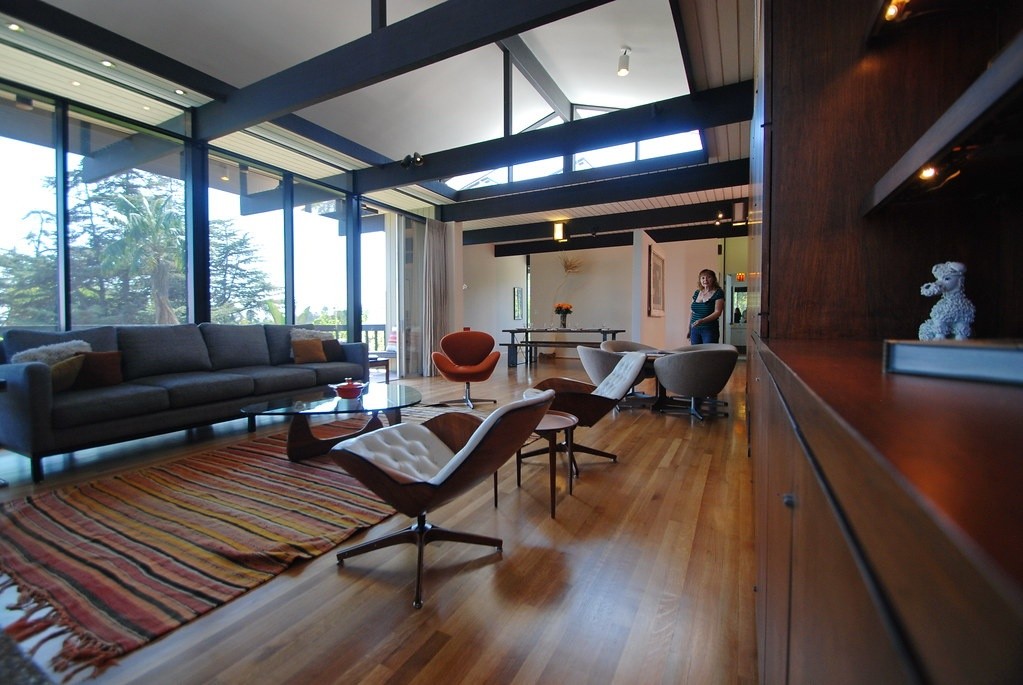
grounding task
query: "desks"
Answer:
[368,358,389,385]
[503,328,626,364]
[632,352,674,414]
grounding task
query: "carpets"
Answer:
[0,407,487,685]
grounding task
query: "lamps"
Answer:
[617,45,632,76]
[553,218,564,241]
[401,152,425,169]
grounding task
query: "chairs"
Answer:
[576,345,644,413]
[654,351,738,421]
[431,327,500,408]
[673,343,737,407]
[521,353,647,479]
[333,389,557,609]
[598,340,660,398]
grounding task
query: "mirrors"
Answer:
[732,286,747,324]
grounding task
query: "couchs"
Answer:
[0,324,369,482]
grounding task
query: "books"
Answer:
[882,338,1023,385]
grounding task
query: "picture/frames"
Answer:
[514,287,523,320]
[649,244,666,317]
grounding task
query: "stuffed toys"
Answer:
[918,261,976,341]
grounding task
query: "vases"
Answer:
[560,314,567,328]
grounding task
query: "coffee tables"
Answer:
[241,385,422,463]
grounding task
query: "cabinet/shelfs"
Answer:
[745,343,916,685]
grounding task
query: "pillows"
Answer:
[321,339,347,362]
[290,339,327,364]
[74,352,121,385]
[51,354,85,395]
[10,339,91,363]
[290,329,334,359]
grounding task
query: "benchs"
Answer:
[498,340,607,348]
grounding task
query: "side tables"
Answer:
[488,411,579,519]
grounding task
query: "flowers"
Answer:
[554,303,572,314]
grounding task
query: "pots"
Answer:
[328,377,369,399]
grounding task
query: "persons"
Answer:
[686,269,725,400]
[734,307,741,323]
